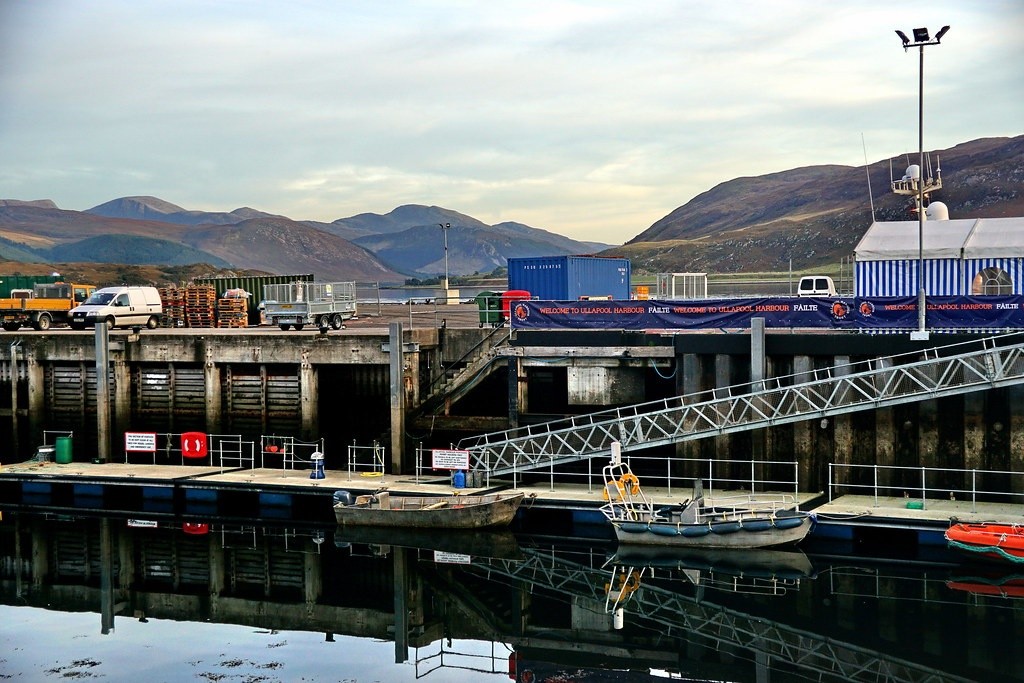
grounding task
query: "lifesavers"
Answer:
[604,582,626,602]
[619,472,640,494]
[620,571,640,592]
[603,480,626,501]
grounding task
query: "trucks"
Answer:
[0,281,99,331]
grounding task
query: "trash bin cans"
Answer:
[476,290,530,328]
[55,437,72,464]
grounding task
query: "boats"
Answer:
[945,576,1024,599]
[331,487,526,529]
[604,543,813,605]
[944,515,1024,562]
[600,462,818,550]
[331,526,524,564]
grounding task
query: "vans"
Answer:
[67,284,163,330]
[797,276,838,298]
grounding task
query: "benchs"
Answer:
[423,501,449,509]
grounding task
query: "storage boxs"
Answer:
[38,445,56,461]
[907,503,923,509]
[465,472,483,487]
[637,286,649,300]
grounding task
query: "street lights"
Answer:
[894,24,952,334]
[439,222,450,291]
